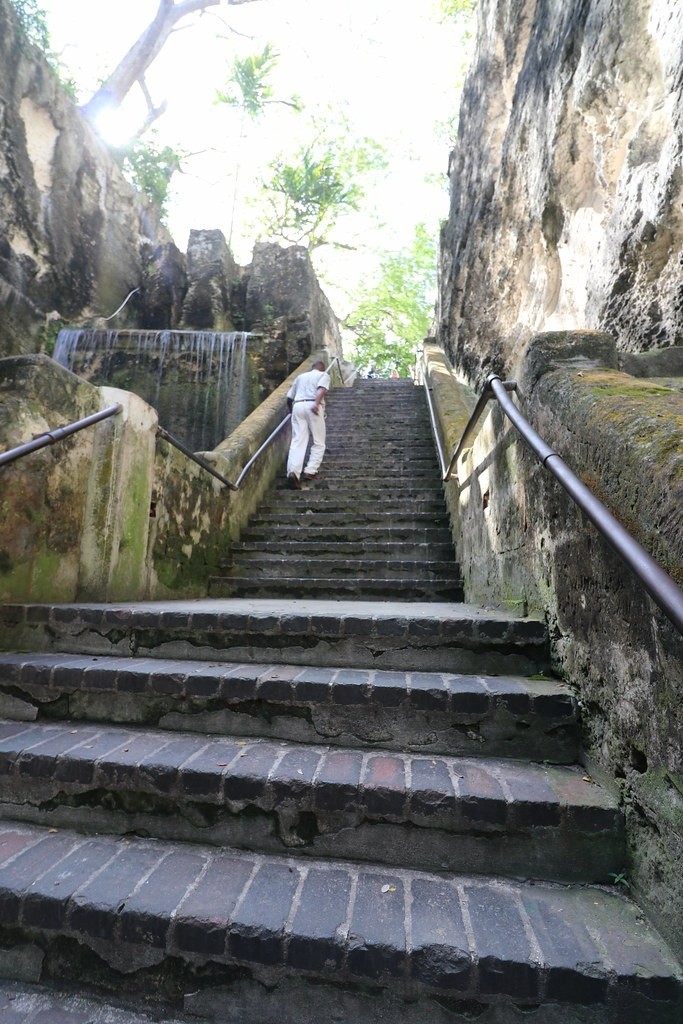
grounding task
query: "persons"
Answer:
[367,360,376,379]
[287,361,331,490]
[389,369,399,379]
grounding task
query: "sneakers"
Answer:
[289,472,301,490]
[303,473,321,481]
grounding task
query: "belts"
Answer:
[295,399,315,402]
